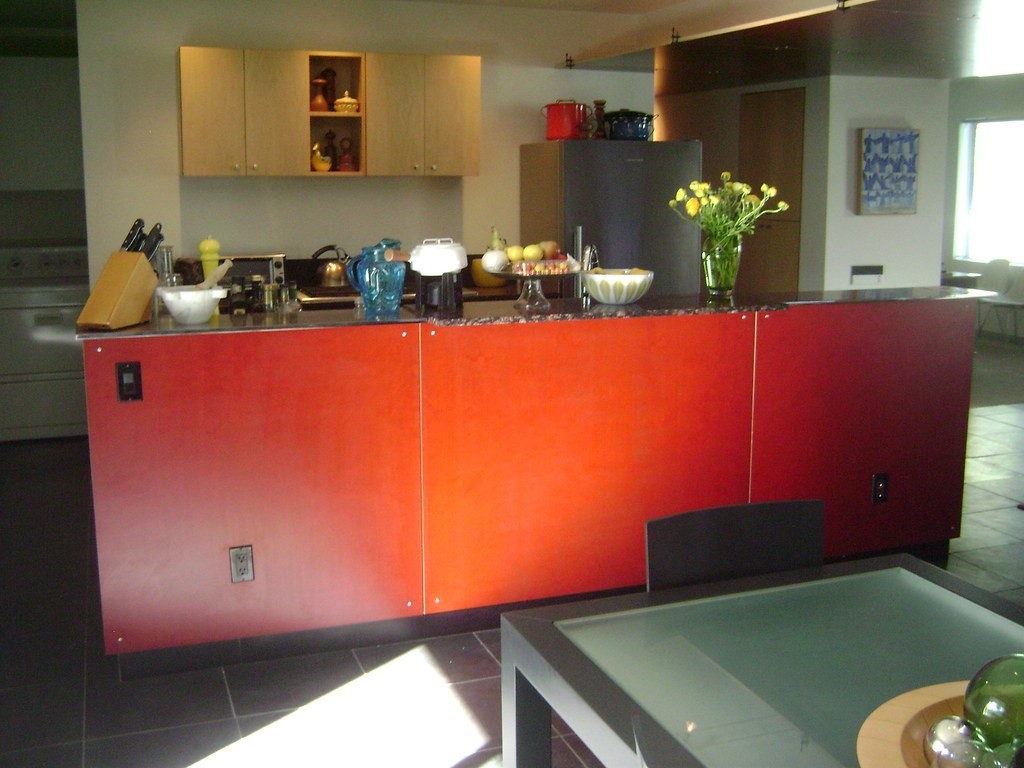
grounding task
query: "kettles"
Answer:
[310,245,353,288]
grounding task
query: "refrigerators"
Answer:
[520,139,703,299]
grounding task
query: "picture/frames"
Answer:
[857,127,921,216]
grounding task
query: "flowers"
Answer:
[669,172,789,286]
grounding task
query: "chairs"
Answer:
[645,500,825,593]
[973,259,1024,343]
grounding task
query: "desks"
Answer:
[501,553,1024,768]
[941,271,981,288]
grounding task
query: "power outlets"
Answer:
[229,544,255,584]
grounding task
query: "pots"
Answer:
[541,99,592,140]
[604,108,657,140]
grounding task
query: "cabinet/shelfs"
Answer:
[179,46,481,177]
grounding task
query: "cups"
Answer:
[253,284,289,308]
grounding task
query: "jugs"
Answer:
[347,238,407,314]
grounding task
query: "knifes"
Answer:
[121,218,164,261]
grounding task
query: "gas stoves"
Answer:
[297,285,477,304]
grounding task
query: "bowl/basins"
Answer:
[581,268,652,305]
[157,283,227,324]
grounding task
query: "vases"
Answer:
[702,234,742,297]
